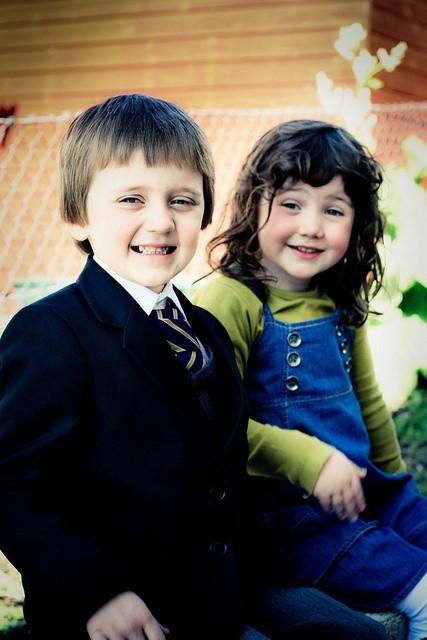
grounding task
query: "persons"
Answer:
[189,118,427,640]
[0,93,390,640]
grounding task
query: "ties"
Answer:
[150,298,208,379]
